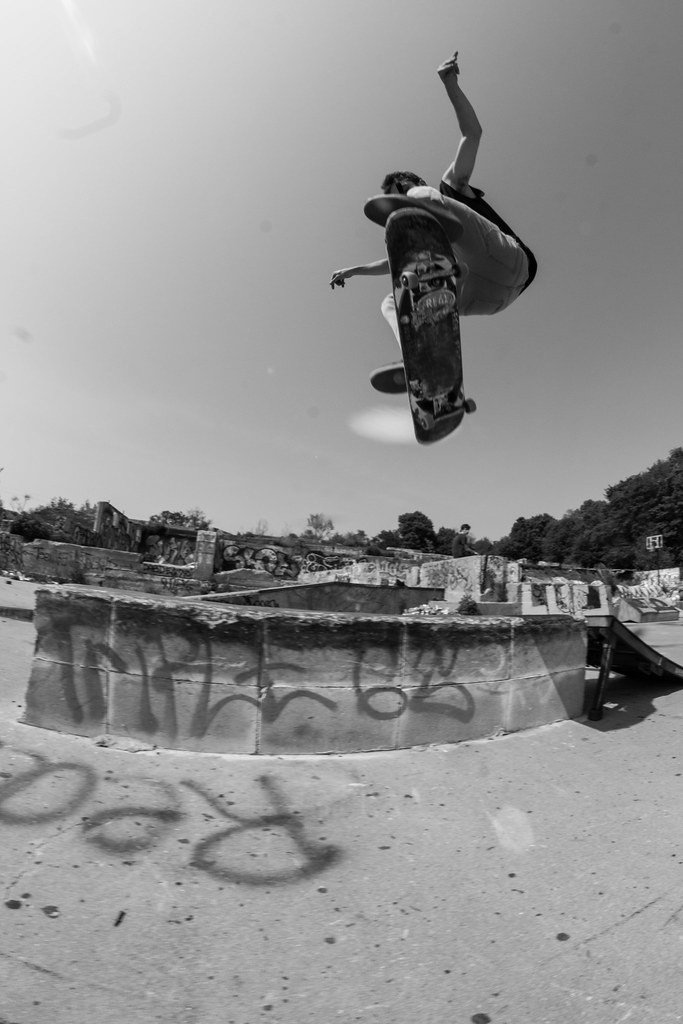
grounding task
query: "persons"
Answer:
[329,51,537,394]
[452,524,477,558]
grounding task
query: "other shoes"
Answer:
[363,194,464,243]
[370,361,406,395]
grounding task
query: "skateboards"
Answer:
[383,203,477,447]
